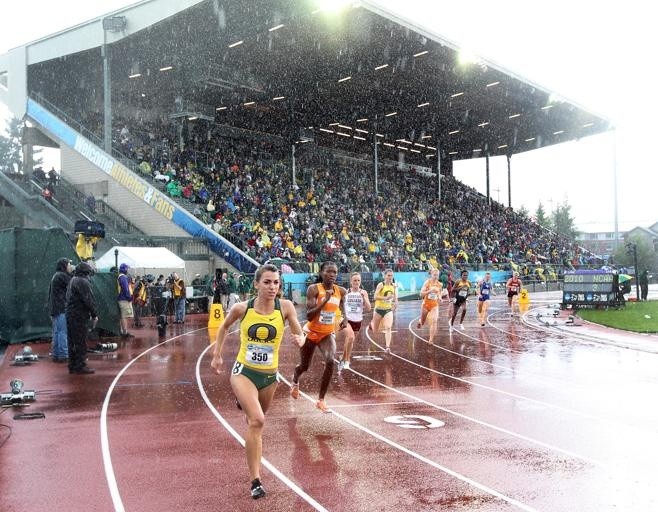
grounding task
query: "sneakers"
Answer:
[248,477,267,501]
[417,312,515,347]
[289,381,301,400]
[315,397,333,415]
[118,319,185,340]
[69,366,94,375]
[337,347,391,373]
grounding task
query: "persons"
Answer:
[640,269,649,299]
[619,281,631,296]
[46,257,249,374]
[290,261,396,413]
[417,268,523,345]
[33,106,628,274]
[209,264,305,499]
